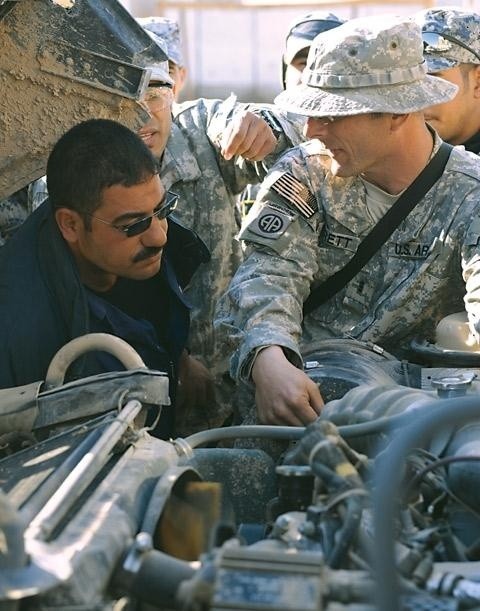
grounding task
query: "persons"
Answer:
[212,15,480,430]
[0,118,211,438]
[134,14,189,102]
[100,30,299,439]
[410,9,480,156]
[260,11,347,94]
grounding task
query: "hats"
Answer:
[284,12,342,67]
[420,8,480,70]
[136,32,176,88]
[272,15,461,121]
[135,16,187,67]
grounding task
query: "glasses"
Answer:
[69,190,182,240]
[422,30,480,64]
[310,117,341,132]
